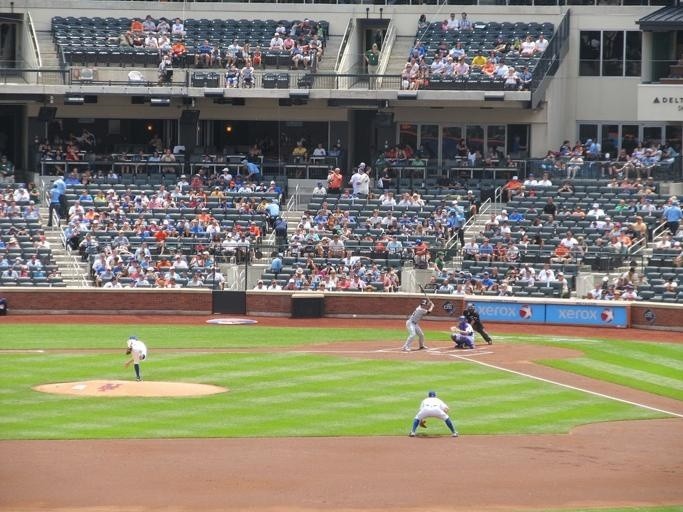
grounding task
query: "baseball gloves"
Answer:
[419,419,427,427]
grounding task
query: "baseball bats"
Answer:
[418,285,432,305]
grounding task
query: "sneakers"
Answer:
[420,346,427,349]
[455,344,463,348]
[489,339,492,344]
[402,348,410,351]
[470,345,474,348]
[137,377,141,381]
[408,432,415,436]
[452,432,458,437]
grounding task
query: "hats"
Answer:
[429,391,435,397]
[130,336,135,339]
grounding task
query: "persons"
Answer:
[268,17,324,70]
[0,298,9,314]
[450,314,475,349]
[461,302,492,345]
[362,43,381,88]
[400,299,435,353]
[118,16,186,85]
[408,389,458,438]
[194,38,263,88]
[402,10,549,90]
[0,128,683,301]
[124,335,147,382]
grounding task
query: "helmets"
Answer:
[459,316,465,321]
[421,299,427,305]
[467,303,474,312]
